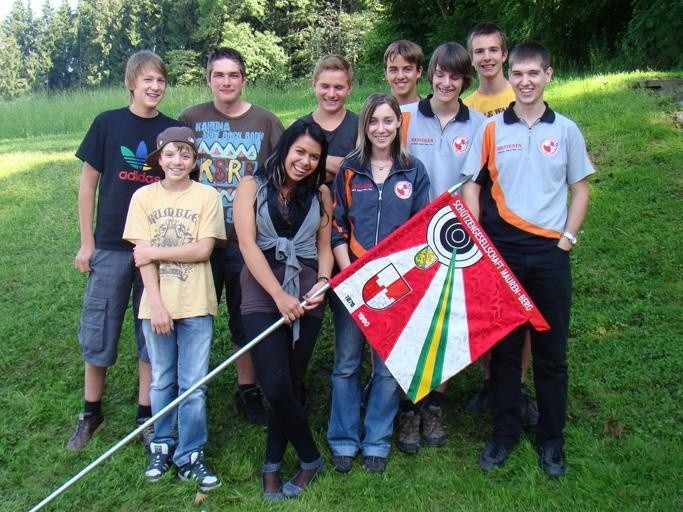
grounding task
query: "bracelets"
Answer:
[315,276,329,282]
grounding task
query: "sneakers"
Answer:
[420,404,446,446]
[538,447,565,476]
[468,377,490,412]
[67,412,105,449]
[263,473,284,501]
[282,461,322,496]
[145,439,174,483]
[521,383,539,428]
[394,409,421,453]
[234,386,271,425]
[177,450,222,491]
[364,456,387,473]
[480,442,509,472]
[331,456,352,473]
[134,417,154,446]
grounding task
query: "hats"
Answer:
[146,126,198,168]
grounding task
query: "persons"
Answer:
[231,119,332,500]
[395,42,485,458]
[328,91,428,475]
[299,55,363,407]
[122,126,224,491]
[461,44,596,481]
[461,23,517,116]
[67,50,189,454]
[174,49,287,423]
[382,39,423,157]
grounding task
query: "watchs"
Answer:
[563,230,577,245]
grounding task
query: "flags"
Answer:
[328,191,550,406]
